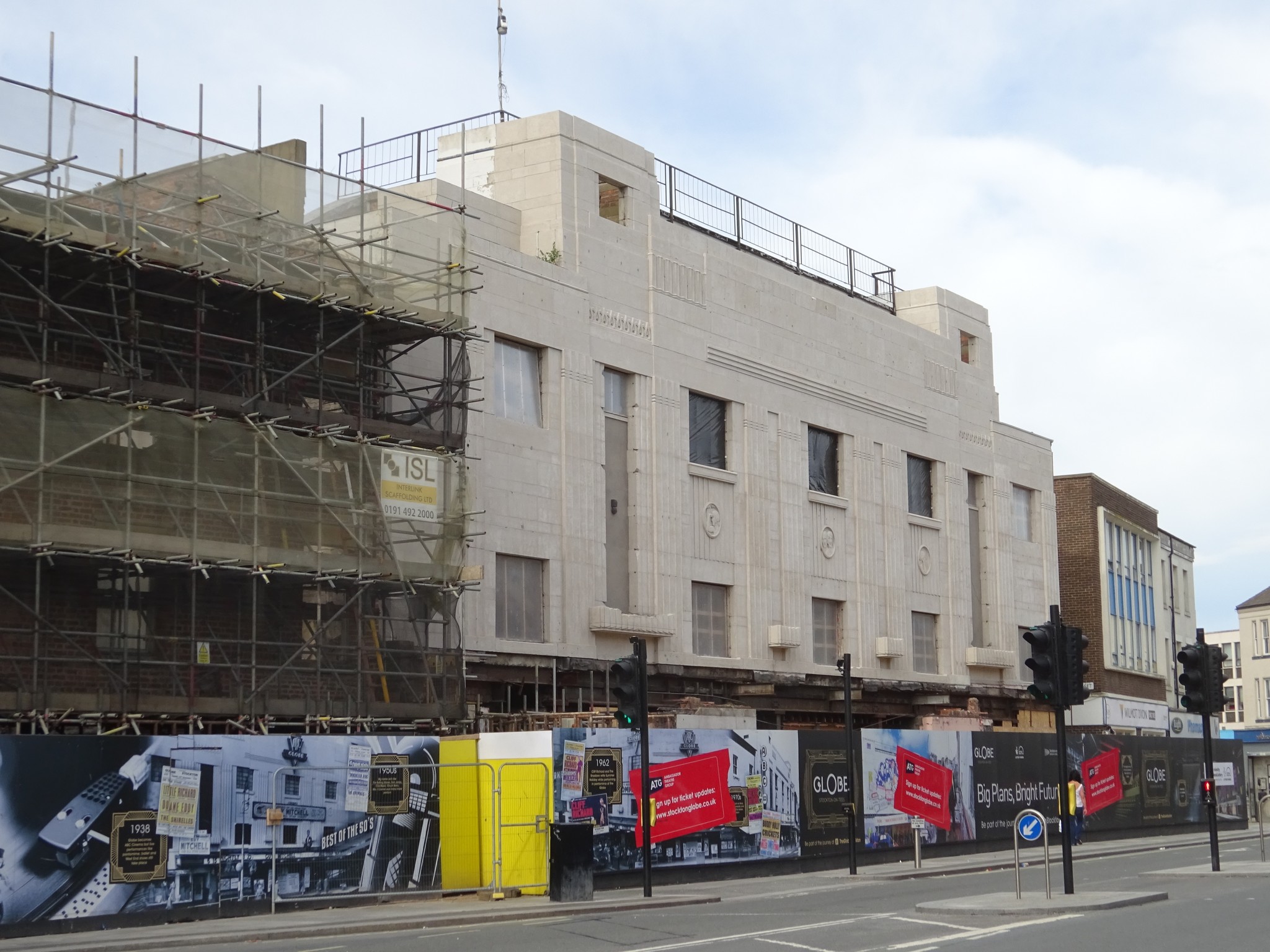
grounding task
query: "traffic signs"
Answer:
[911,819,925,829]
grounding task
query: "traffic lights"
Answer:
[1177,645,1206,714]
[1206,646,1228,713]
[1060,626,1091,708]
[1023,623,1061,707]
[612,655,641,728]
[1201,780,1215,806]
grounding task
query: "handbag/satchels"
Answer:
[1059,820,1062,833]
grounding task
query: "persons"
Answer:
[1068,769,1087,846]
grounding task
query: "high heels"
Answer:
[1071,835,1083,846]
